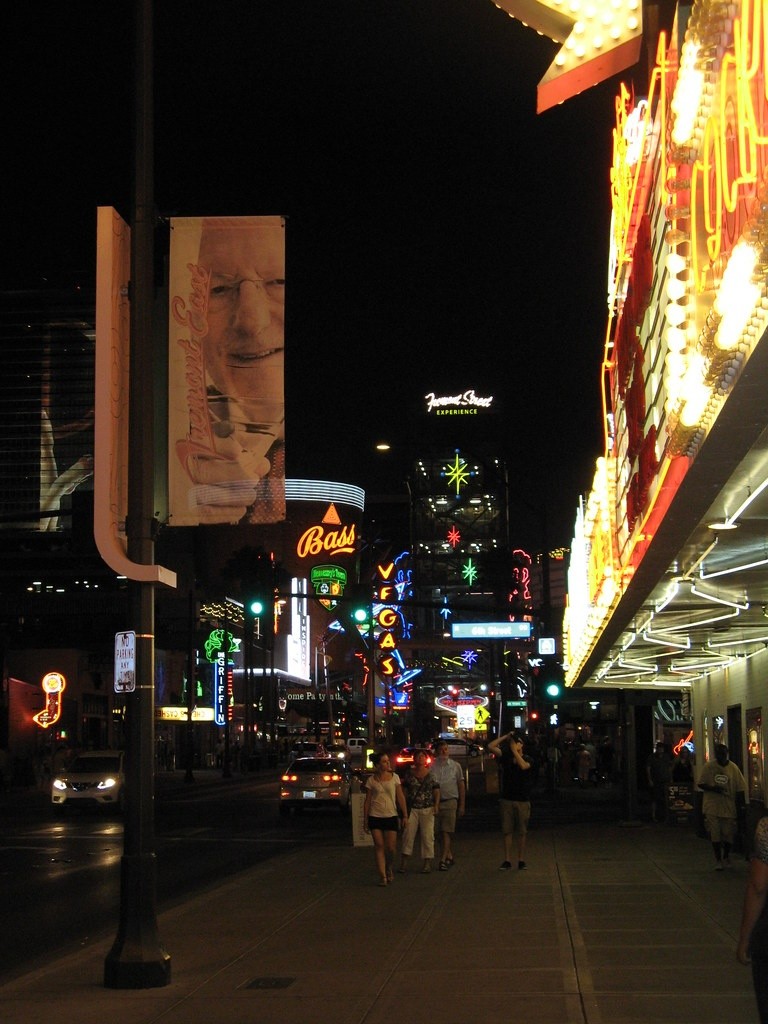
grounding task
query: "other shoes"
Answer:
[714,860,723,870]
[519,861,527,870]
[724,857,732,868]
[499,861,512,870]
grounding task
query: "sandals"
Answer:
[439,857,454,870]
[422,859,433,873]
[398,859,408,872]
[381,875,387,886]
[387,866,394,881]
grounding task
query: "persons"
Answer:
[532,733,618,788]
[428,740,466,871]
[213,738,298,773]
[185,215,285,526]
[364,752,408,886]
[673,746,694,784]
[646,742,673,823]
[736,816,768,1024]
[698,744,748,871]
[396,749,440,873]
[487,730,534,870]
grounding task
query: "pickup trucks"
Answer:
[346,737,370,756]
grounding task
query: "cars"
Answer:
[325,744,351,760]
[52,750,127,817]
[433,738,485,758]
[394,748,434,775]
[279,757,360,811]
[287,742,329,767]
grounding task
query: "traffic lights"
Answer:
[549,712,559,728]
[246,570,270,616]
[350,584,370,623]
[544,682,562,699]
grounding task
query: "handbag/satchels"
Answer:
[399,800,412,819]
